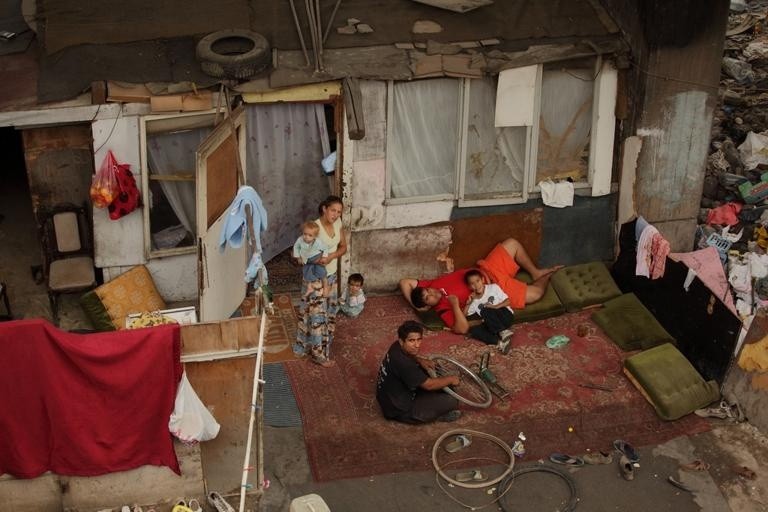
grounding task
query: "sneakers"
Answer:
[498,329,514,342]
[721,391,745,422]
[584,448,613,465]
[619,454,634,480]
[174,499,202,512]
[496,341,511,354]
[669,476,695,491]
[207,491,235,512]
[695,408,726,418]
[436,410,461,421]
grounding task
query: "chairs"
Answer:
[34,202,98,326]
[0,283,13,319]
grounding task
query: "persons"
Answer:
[291,218,334,300]
[376,320,464,424]
[461,269,517,354]
[294,194,346,367]
[398,237,568,334]
[335,274,368,319]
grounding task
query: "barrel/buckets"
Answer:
[290,493,331,512]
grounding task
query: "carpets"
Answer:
[241,293,305,364]
[286,294,712,482]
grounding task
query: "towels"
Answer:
[636,217,670,279]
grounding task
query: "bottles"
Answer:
[480,366,497,385]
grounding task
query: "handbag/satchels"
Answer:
[108,151,142,220]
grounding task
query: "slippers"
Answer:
[682,461,709,471]
[445,435,472,452]
[613,440,640,462]
[730,465,757,480]
[550,453,584,467]
[455,470,488,482]
